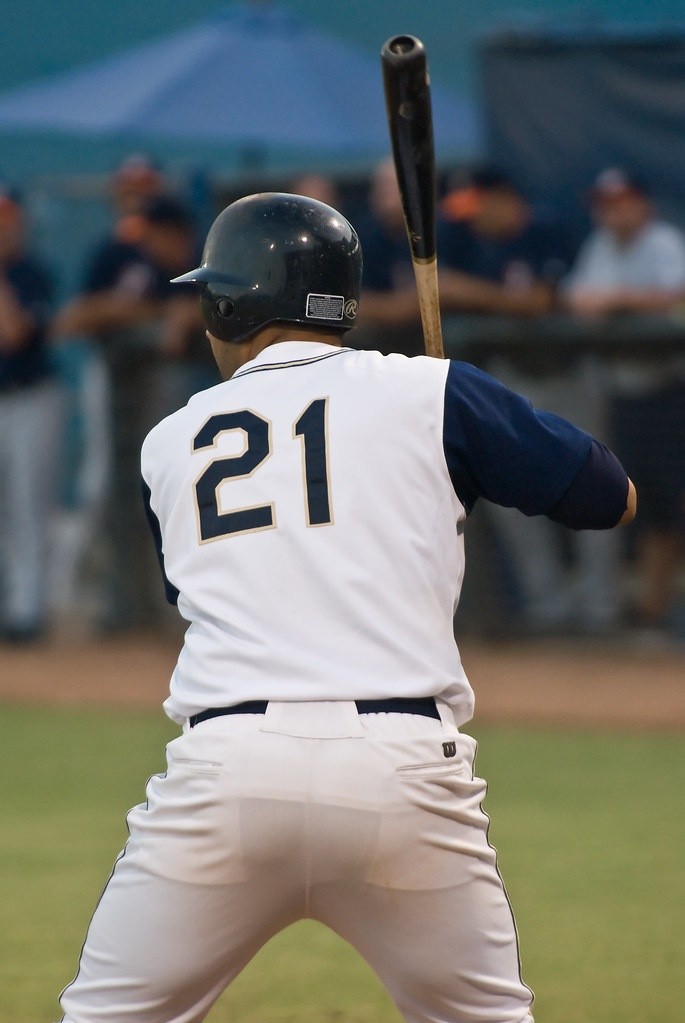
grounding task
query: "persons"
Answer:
[59,190,637,1023]
[1,157,685,636]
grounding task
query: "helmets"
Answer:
[170,193,364,344]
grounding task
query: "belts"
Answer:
[189,696,441,728]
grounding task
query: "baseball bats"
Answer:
[380,35,443,360]
